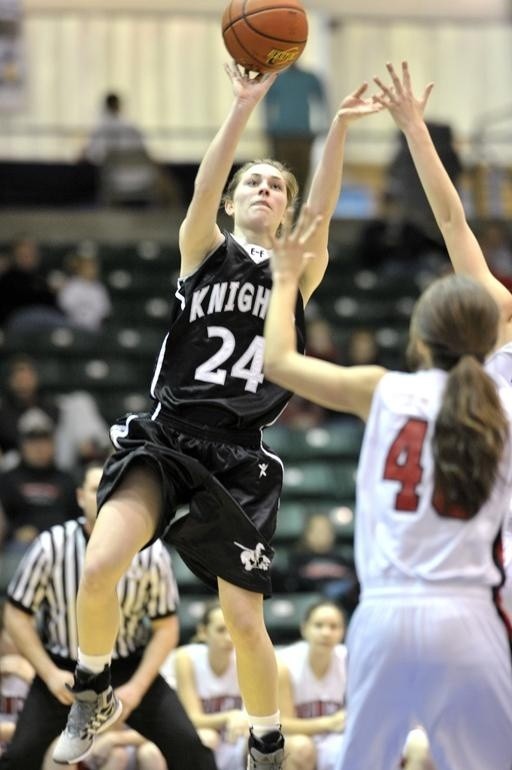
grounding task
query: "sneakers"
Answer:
[49,661,124,766]
[244,726,287,770]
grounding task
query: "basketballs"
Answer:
[222,0,308,73]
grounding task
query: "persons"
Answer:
[256,62,332,221]
[379,117,461,224]
[263,205,511,768]
[84,91,154,212]
[2,459,215,768]
[2,222,511,621]
[1,603,431,768]
[53,61,394,769]
[373,62,512,619]
